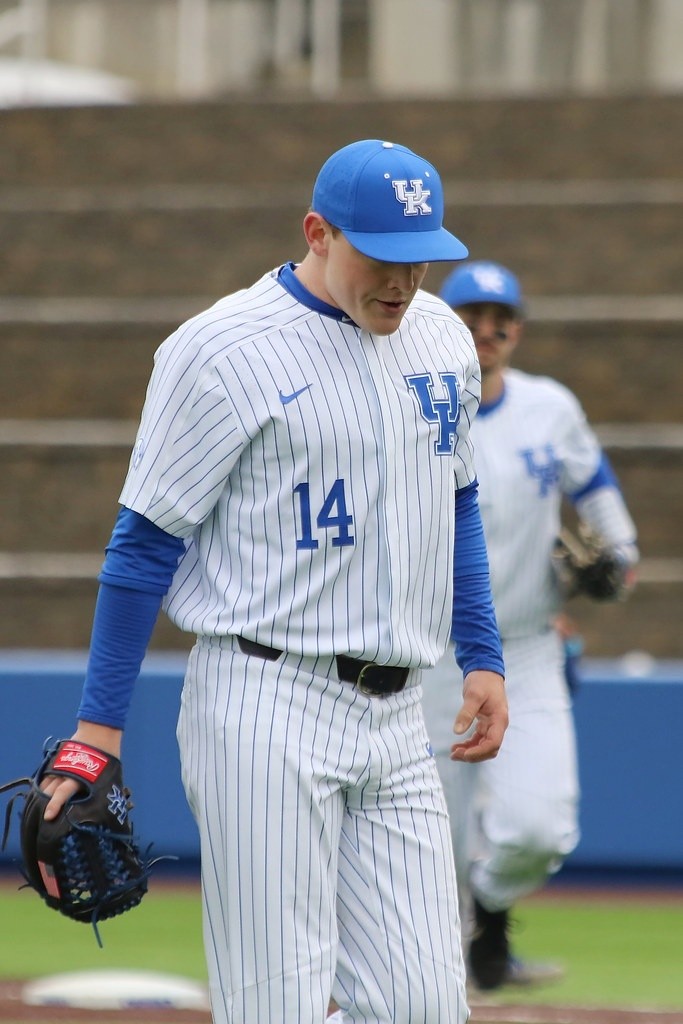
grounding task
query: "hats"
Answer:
[439,261,522,307]
[311,139,469,263]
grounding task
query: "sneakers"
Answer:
[469,893,510,988]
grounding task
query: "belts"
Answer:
[236,634,409,696]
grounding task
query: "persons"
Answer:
[422,262,641,993]
[19,137,509,1023]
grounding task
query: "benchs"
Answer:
[1,93,683,671]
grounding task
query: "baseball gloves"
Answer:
[18,737,151,925]
